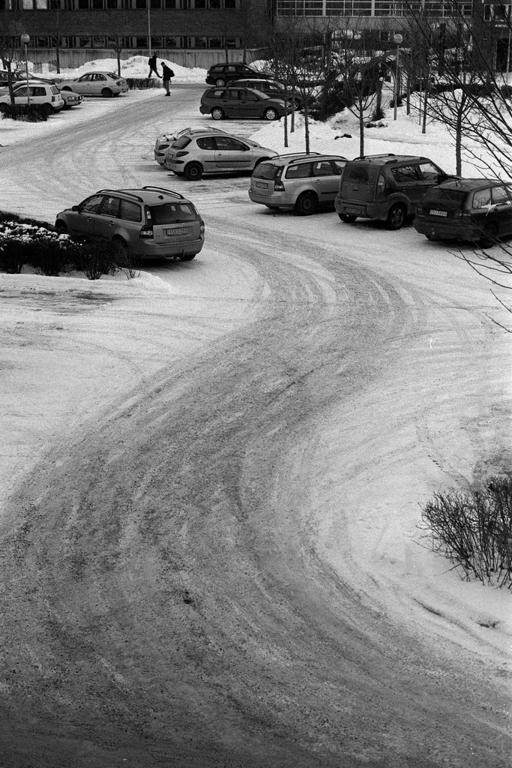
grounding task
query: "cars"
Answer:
[200,62,315,120]
[0,70,129,116]
[55,186,205,265]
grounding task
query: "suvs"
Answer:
[153,125,261,171]
[412,178,512,250]
[248,152,349,216]
[334,153,465,231]
[165,132,280,181]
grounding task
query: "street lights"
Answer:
[393,34,403,120]
[21,33,30,106]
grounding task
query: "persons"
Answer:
[148,52,163,78]
[161,62,175,96]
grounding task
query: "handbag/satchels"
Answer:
[148,58,152,65]
[170,70,173,77]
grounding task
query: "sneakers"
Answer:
[165,92,170,96]
